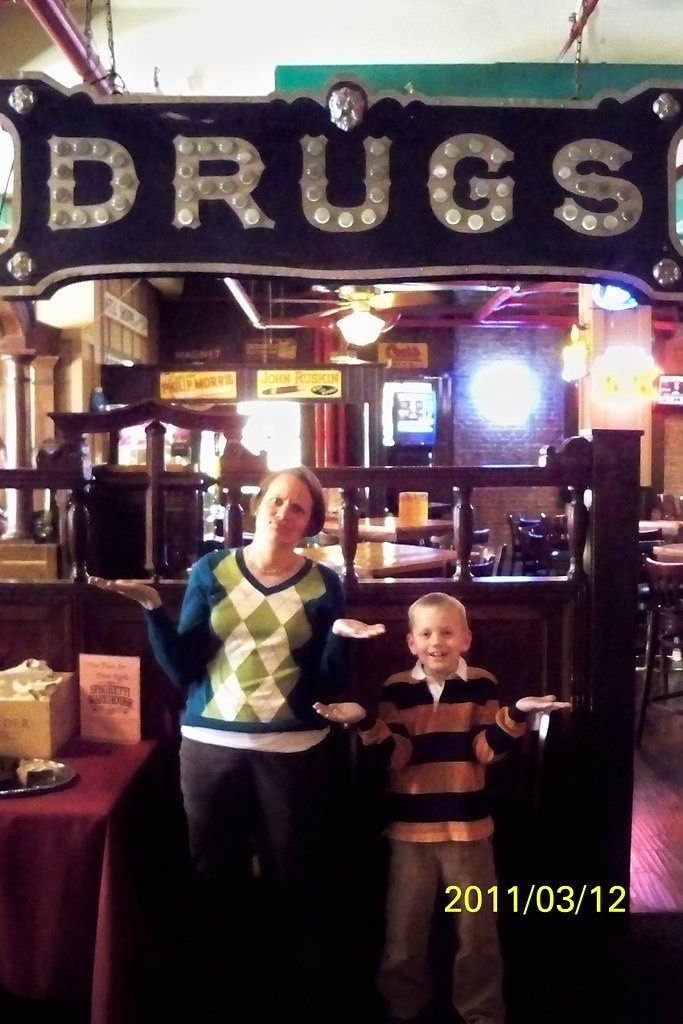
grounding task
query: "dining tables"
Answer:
[0,732,161,1021]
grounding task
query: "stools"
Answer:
[638,557,683,750]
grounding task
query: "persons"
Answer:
[87,463,385,949]
[312,592,572,1024]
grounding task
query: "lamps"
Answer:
[32,280,95,328]
[334,300,386,346]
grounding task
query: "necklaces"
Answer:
[246,547,296,574]
